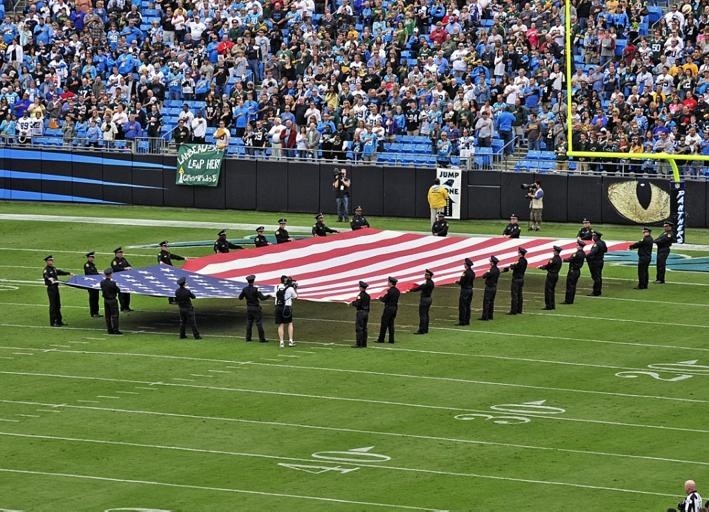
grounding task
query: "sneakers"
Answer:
[351,344,368,349]
[280,339,285,348]
[652,279,665,284]
[120,307,134,312]
[107,329,124,334]
[194,336,203,340]
[633,285,648,289]
[258,338,270,343]
[528,226,542,231]
[373,339,395,344]
[90,313,104,318]
[477,318,494,321]
[587,291,602,296]
[335,216,350,223]
[505,310,523,316]
[50,319,68,328]
[540,305,556,311]
[246,339,254,341]
[179,335,188,339]
[169,300,178,305]
[288,339,297,348]
[413,329,429,335]
[558,300,574,305]
[453,322,470,327]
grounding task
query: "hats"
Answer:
[552,245,563,253]
[103,267,113,275]
[488,255,500,264]
[176,276,186,286]
[353,206,363,211]
[643,227,653,232]
[277,218,287,224]
[315,212,324,219]
[518,247,528,254]
[437,212,445,217]
[112,246,124,253]
[217,229,228,236]
[158,240,170,246]
[664,220,675,228]
[591,230,604,237]
[581,217,591,224]
[246,274,256,282]
[424,269,434,276]
[359,280,369,288]
[42,255,55,262]
[576,240,585,247]
[464,257,474,268]
[255,226,265,232]
[510,213,521,219]
[84,250,97,257]
[388,276,399,284]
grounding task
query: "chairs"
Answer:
[0,0,709,181]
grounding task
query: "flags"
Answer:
[172,144,225,188]
[61,225,640,306]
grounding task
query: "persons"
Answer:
[452,258,476,327]
[576,216,596,241]
[273,275,299,349]
[253,226,273,248]
[350,204,370,231]
[212,229,248,255]
[99,268,123,335]
[427,178,451,230]
[559,240,586,305]
[476,255,501,321]
[678,479,703,512]
[174,276,203,341]
[238,274,272,343]
[505,246,529,315]
[348,280,371,349]
[111,246,135,312]
[627,227,654,290]
[373,275,401,344]
[83,250,104,319]
[536,245,563,310]
[431,211,449,237]
[311,212,340,238]
[332,167,352,224]
[653,220,674,285]
[274,218,296,244]
[406,268,435,335]
[157,239,188,305]
[527,179,546,233]
[585,231,608,297]
[42,254,76,328]
[501,213,522,273]
